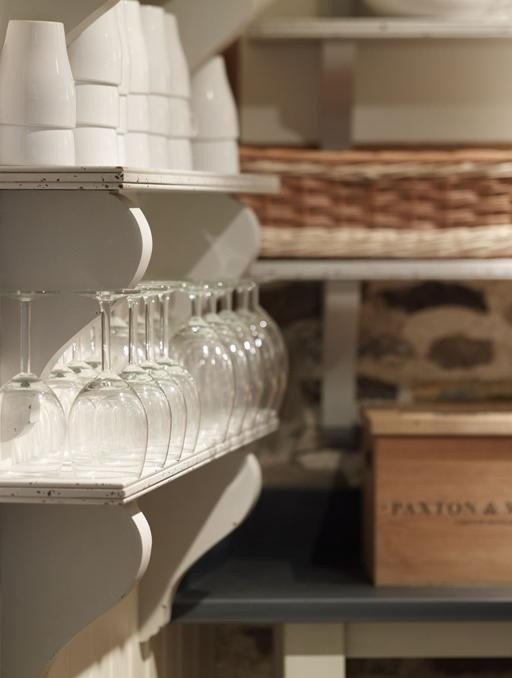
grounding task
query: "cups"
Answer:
[0,0,242,173]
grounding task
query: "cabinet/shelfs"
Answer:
[1,2,280,678]
[239,17,512,443]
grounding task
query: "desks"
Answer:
[175,484,511,678]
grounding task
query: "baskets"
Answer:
[237,139,512,260]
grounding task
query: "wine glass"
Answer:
[0,279,292,480]
